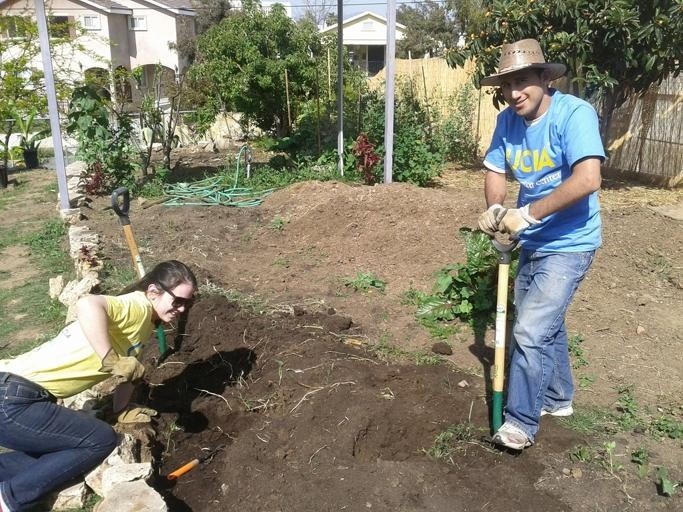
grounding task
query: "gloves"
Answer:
[98,346,146,384]
[113,402,158,423]
[498,203,542,237]
[477,203,508,237]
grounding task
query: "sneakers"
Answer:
[491,419,532,450]
[540,403,574,417]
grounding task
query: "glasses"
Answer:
[158,282,195,309]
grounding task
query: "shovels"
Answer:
[111,187,175,367]
[481,233,516,445]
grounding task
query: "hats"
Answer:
[479,38,567,86]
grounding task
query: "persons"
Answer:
[477,37,606,451]
[0,260,198,512]
